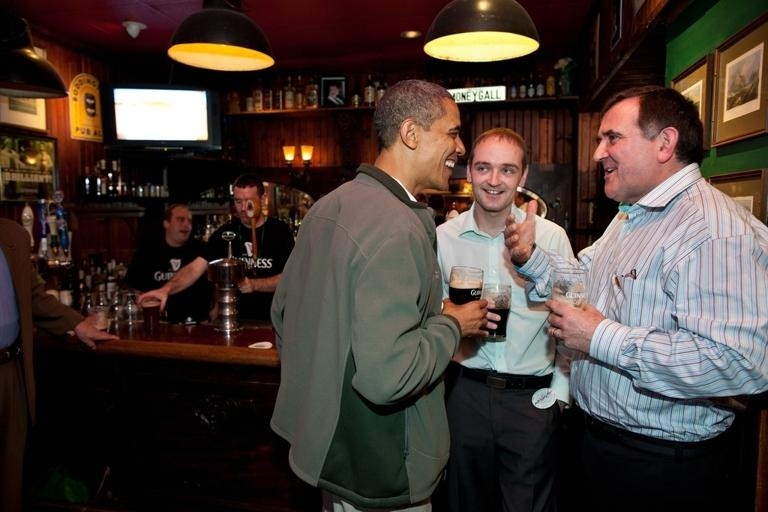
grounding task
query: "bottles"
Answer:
[83,159,121,196]
[252,88,283,111]
[122,180,165,196]
[285,74,304,109]
[349,74,387,106]
[76,255,137,317]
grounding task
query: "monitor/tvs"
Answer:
[100,84,220,149]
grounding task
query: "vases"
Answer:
[560,80,570,96]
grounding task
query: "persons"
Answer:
[127,205,214,321]
[507,86,767,511]
[270,78,466,511]
[138,173,294,323]
[1,216,120,512]
[434,128,576,512]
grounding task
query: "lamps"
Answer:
[0,13,71,100]
[166,0,275,73]
[282,146,296,185]
[423,0,541,65]
[301,145,315,183]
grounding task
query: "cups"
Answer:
[553,268,588,311]
[449,266,483,305]
[141,300,161,338]
[481,284,512,343]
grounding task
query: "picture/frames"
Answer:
[0,44,48,133]
[587,1,629,102]
[670,53,714,153]
[0,126,62,205]
[630,1,668,44]
[710,9,768,149]
[706,168,768,228]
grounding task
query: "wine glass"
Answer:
[109,293,140,327]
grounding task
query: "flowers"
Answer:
[555,58,575,81]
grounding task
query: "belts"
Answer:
[602,427,689,462]
[469,369,553,390]
[0,343,21,365]
